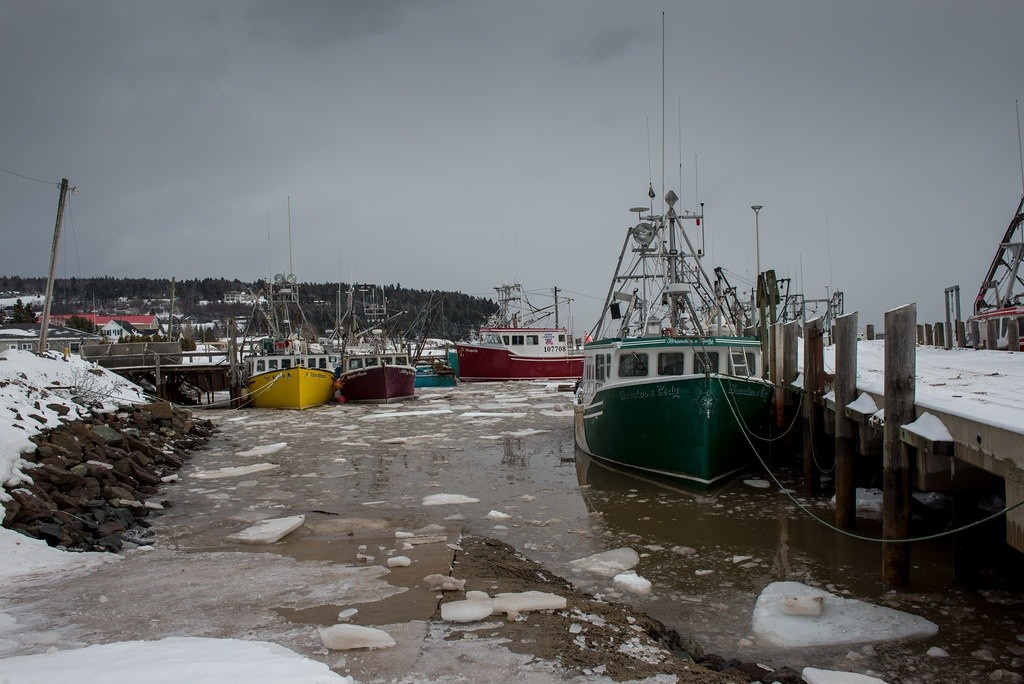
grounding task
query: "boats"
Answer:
[570,184,775,494]
[453,283,593,381]
[332,280,442,406]
[232,270,335,410]
